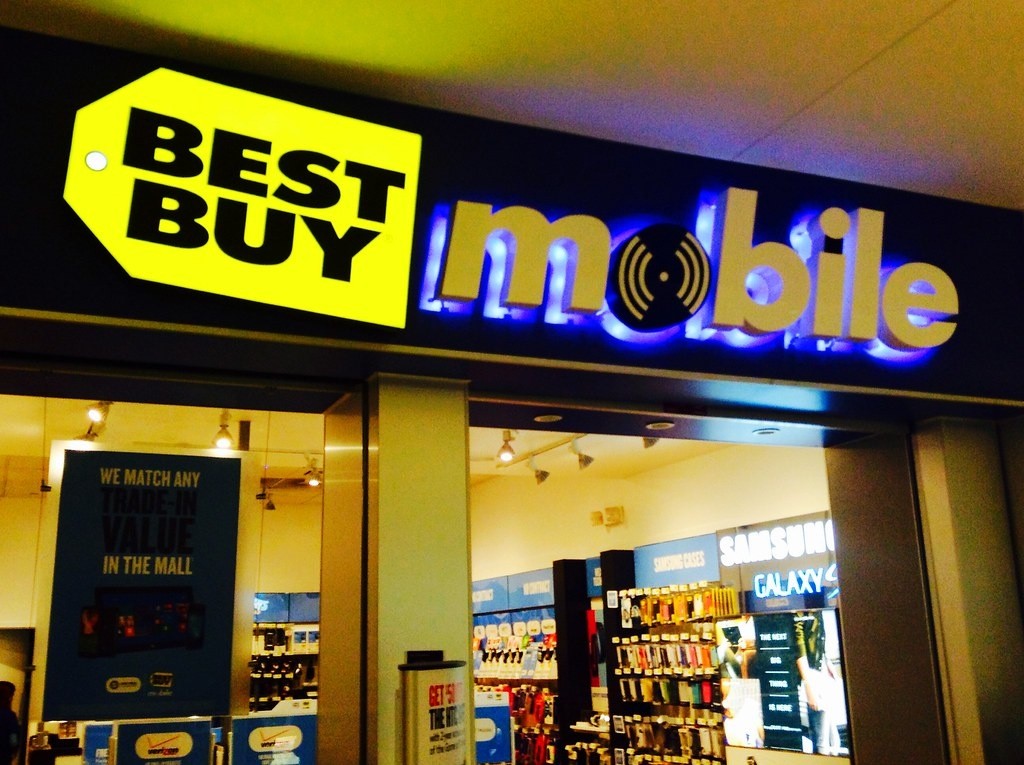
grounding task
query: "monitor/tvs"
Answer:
[254,592,321,623]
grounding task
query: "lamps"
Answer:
[87,401,114,422]
[49,431,101,485]
[526,456,550,486]
[568,439,595,471]
[496,430,516,462]
[212,409,234,449]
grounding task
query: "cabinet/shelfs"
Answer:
[247,606,558,713]
